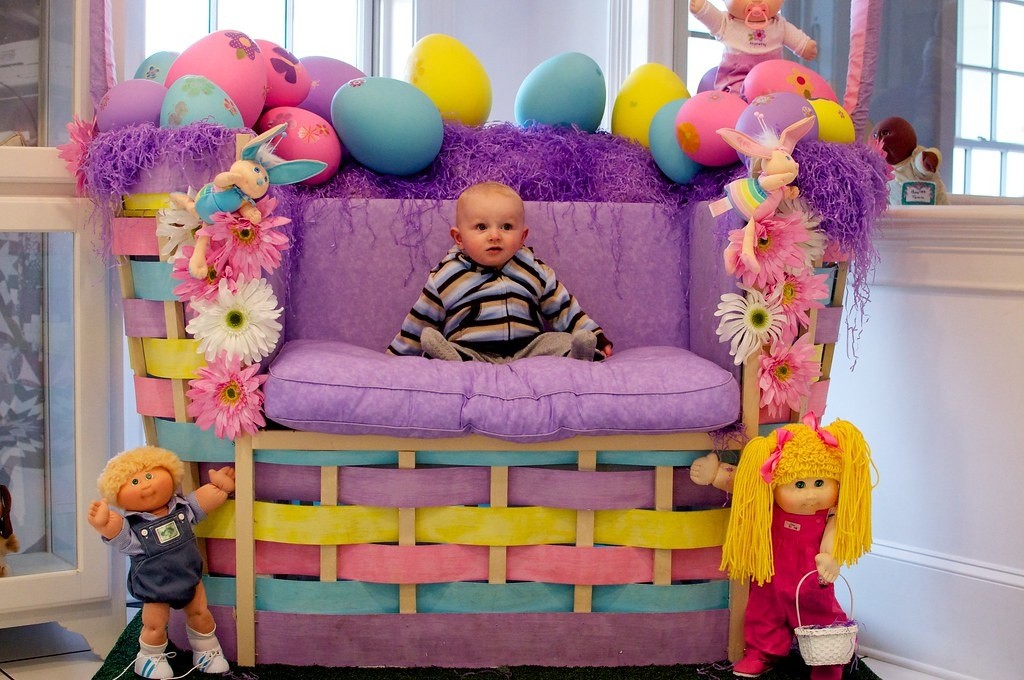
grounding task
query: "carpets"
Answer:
[91,610,888,680]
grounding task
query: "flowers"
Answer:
[715,206,823,419]
[54,114,96,192]
[156,188,292,439]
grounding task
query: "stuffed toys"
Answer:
[87,447,236,678]
[869,118,952,206]
[709,118,816,228]
[689,1,821,97]
[174,121,332,281]
[692,421,883,680]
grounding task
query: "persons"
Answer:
[387,184,615,362]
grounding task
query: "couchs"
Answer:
[84,111,900,665]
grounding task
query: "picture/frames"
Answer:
[1,193,114,615]
[0,1,91,185]
